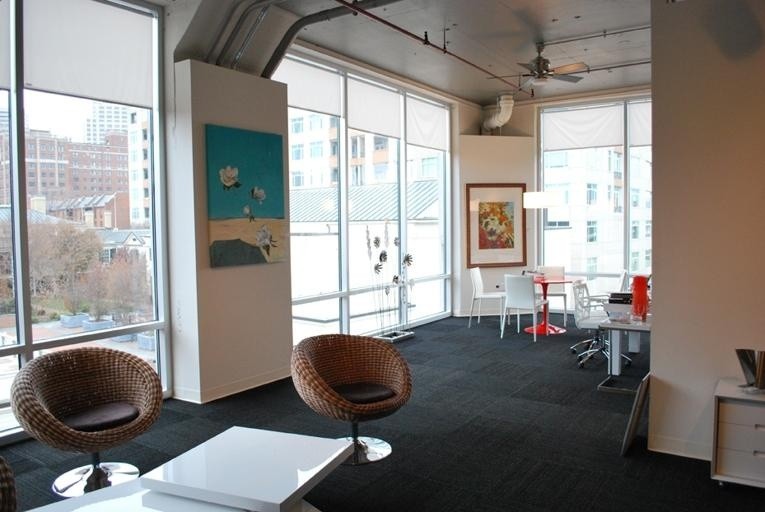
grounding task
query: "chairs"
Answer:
[0,455,16,512]
[9,346,163,498]
[468,265,632,370]
[291,333,412,466]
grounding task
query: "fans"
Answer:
[485,42,590,97]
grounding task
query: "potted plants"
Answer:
[55,248,156,350]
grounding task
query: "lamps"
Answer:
[523,190,564,265]
[532,78,548,85]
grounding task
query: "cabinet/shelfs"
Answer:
[710,377,765,488]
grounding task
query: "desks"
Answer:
[599,314,651,376]
[23,425,354,512]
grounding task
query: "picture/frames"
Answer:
[466,183,527,269]
